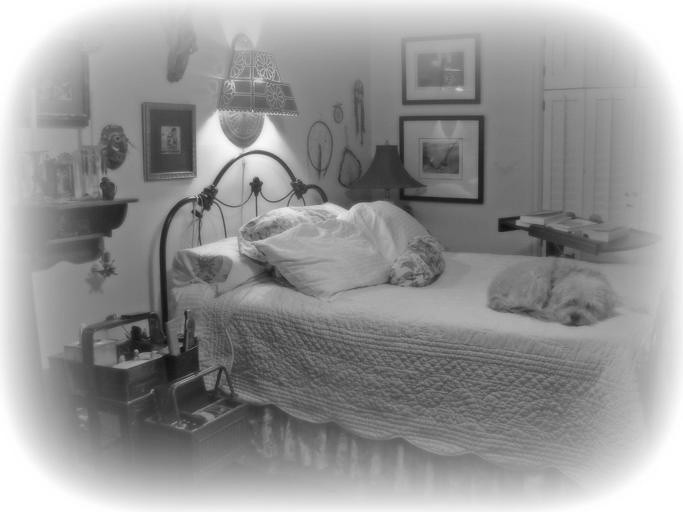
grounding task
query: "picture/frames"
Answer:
[140,100,197,183]
[397,32,485,207]
[35,50,91,129]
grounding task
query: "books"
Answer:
[516,210,627,242]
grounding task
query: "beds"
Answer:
[158,148,666,489]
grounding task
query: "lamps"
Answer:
[214,31,300,120]
[348,140,428,202]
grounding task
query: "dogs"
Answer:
[486,256,624,326]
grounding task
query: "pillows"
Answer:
[170,236,268,297]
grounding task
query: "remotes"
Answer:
[184,310,195,351]
[148,313,163,344]
[165,319,181,356]
